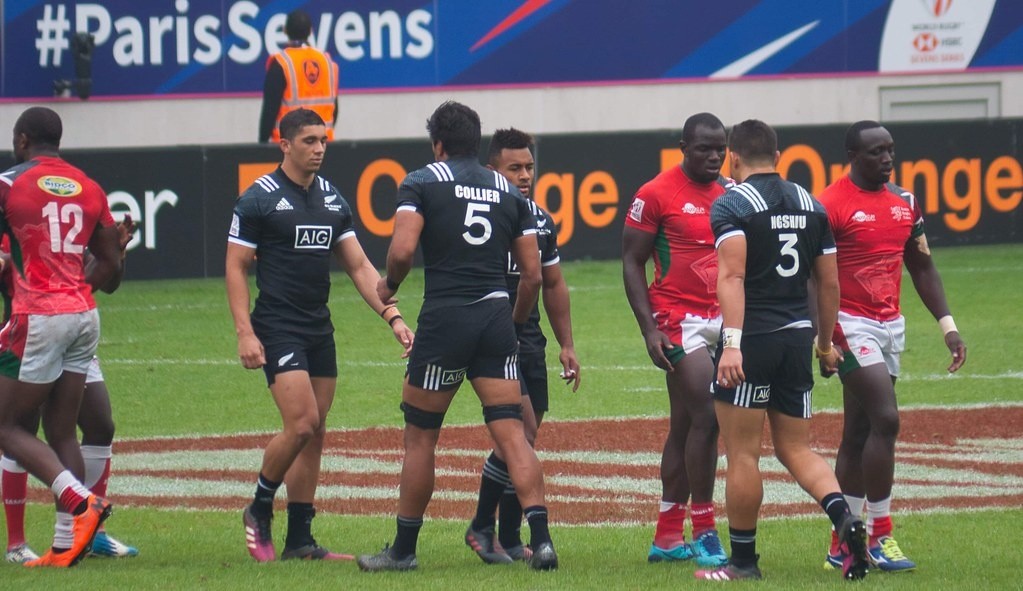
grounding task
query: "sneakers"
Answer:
[694,553,761,582]
[866,534,916,574]
[21,542,89,567]
[834,513,869,582]
[692,528,727,568]
[464,519,513,566]
[645,539,699,563]
[280,537,354,562]
[528,541,558,572]
[243,505,276,564]
[357,542,417,572]
[3,546,38,565]
[89,532,138,558]
[824,547,885,573]
[60,494,112,567]
[502,540,532,560]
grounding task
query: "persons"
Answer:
[622,114,740,568]
[224,110,413,564]
[693,118,868,579]
[0,105,139,572]
[256,8,342,141]
[805,117,965,574]
[357,97,561,571]
[463,127,581,565]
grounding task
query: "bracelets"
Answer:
[513,321,526,336]
[815,343,833,355]
[812,333,834,346]
[380,304,404,326]
[938,314,959,336]
[721,328,743,350]
